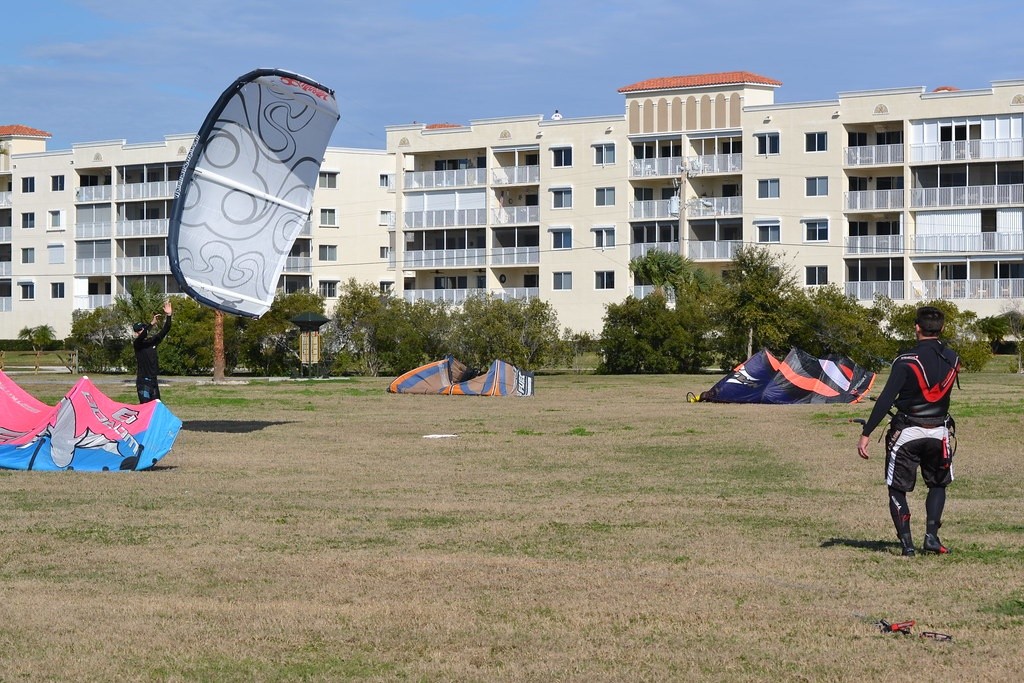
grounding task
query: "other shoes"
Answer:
[920,533,951,554]
[898,533,916,557]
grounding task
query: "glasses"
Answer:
[138,325,147,331]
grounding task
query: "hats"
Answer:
[133,322,147,331]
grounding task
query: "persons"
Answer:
[551,110,563,120]
[858,307,960,556]
[133,301,172,404]
